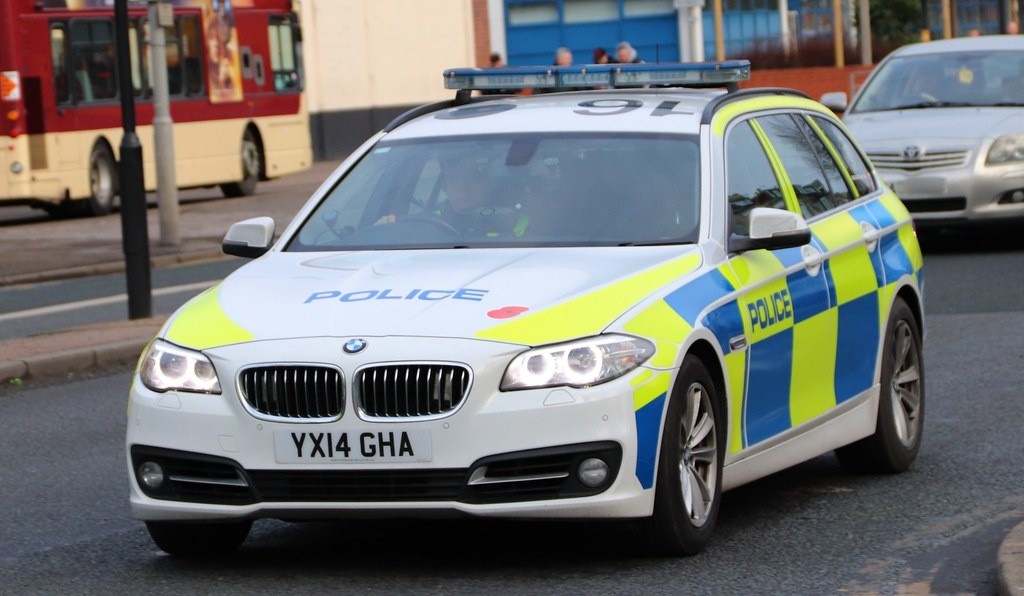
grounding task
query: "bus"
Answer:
[0,0,315,217]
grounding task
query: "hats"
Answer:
[593,48,605,61]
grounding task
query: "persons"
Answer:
[594,41,657,89]
[541,47,594,93]
[968,28,981,36]
[1006,21,1020,34]
[482,54,522,94]
[372,155,541,239]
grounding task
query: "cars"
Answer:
[821,34,1023,251]
[122,59,928,561]
